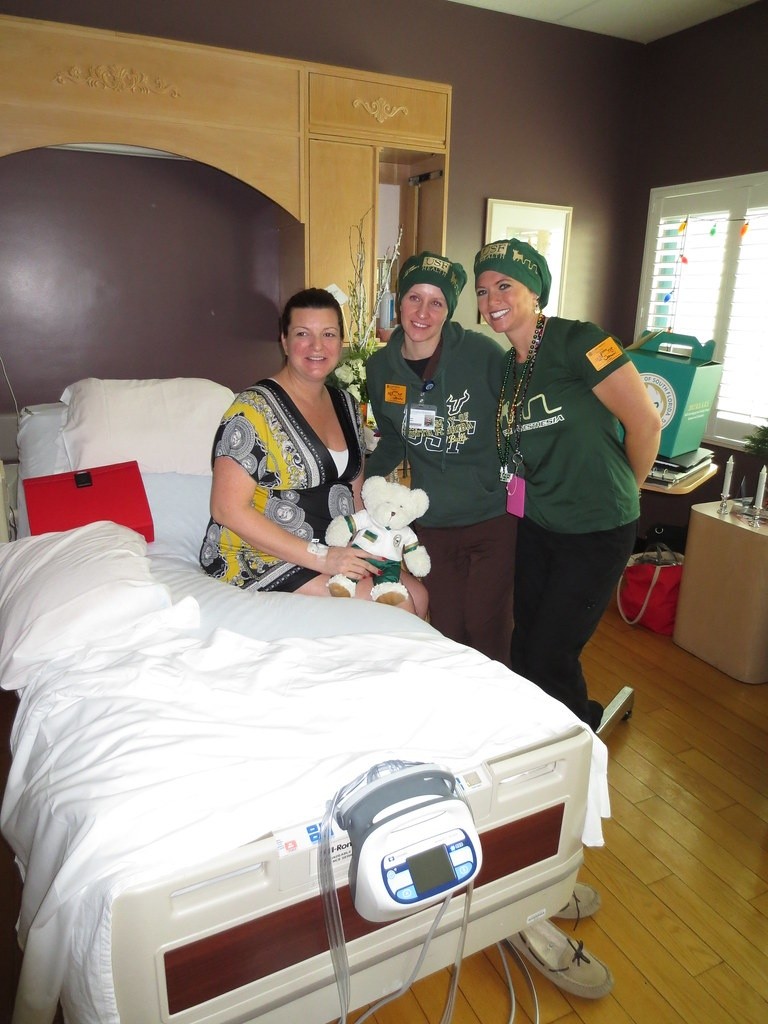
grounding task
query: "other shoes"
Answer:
[506,919,614,999]
[553,882,603,918]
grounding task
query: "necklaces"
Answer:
[496,310,546,481]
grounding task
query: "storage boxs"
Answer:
[23,460,155,542]
[618,329,723,459]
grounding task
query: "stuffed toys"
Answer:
[325,476,431,607]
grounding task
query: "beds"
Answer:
[0,393,591,1024]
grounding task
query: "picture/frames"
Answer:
[479,198,574,324]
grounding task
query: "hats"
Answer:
[474,238,552,310]
[395,251,468,322]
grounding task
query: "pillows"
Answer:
[61,377,236,476]
[1,520,166,691]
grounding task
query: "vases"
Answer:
[360,403,367,424]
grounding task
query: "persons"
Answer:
[363,252,519,668]
[474,237,661,737]
[199,287,428,620]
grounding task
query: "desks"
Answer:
[672,497,768,684]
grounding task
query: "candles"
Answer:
[755,465,767,508]
[723,455,734,496]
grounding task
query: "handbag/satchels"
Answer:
[616,551,685,636]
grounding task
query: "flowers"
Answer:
[334,205,404,404]
